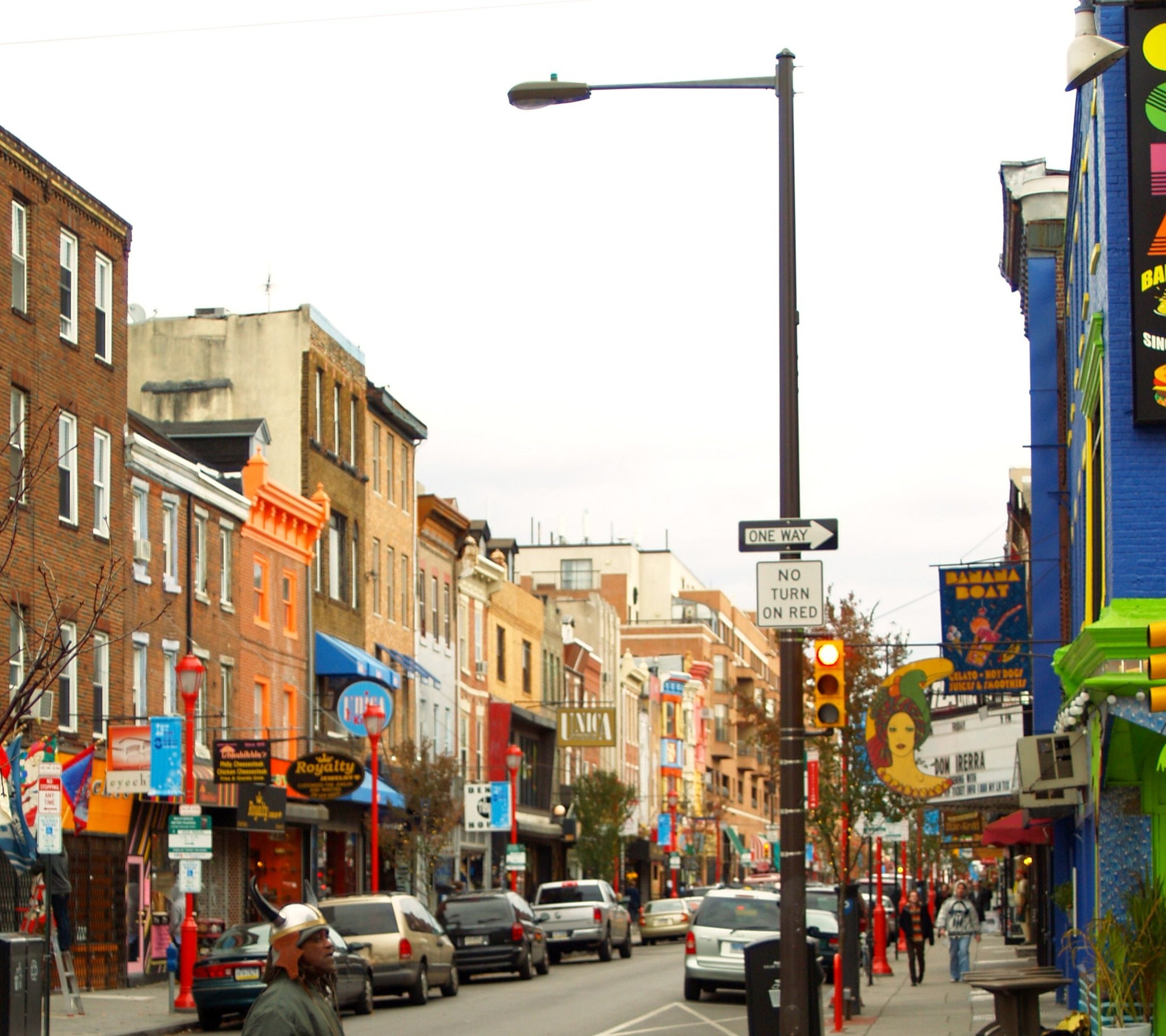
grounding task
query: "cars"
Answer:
[682,870,920,985]
[191,920,377,1032]
[638,897,693,947]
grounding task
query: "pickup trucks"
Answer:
[528,879,633,966]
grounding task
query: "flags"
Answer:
[0,733,96,877]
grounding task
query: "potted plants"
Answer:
[1060,896,1151,1036]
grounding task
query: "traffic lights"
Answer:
[813,639,846,729]
[762,842,770,859]
[1146,618,1166,713]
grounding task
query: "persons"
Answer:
[240,874,346,1036]
[31,844,71,952]
[900,878,994,987]
[1013,868,1032,946]
[170,882,186,983]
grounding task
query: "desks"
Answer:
[962,966,1072,1036]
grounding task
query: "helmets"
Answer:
[247,875,330,946]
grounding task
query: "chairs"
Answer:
[756,908,778,919]
[722,906,737,923]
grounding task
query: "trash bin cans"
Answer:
[744,934,824,1036]
[1,931,47,1036]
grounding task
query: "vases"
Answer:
[1021,923,1036,944]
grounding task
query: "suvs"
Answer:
[314,890,460,1007]
[433,886,551,985]
[683,889,782,1002]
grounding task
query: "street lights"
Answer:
[362,699,388,896]
[172,651,208,1017]
[504,46,804,1036]
[666,788,680,899]
[711,802,724,884]
[503,742,524,892]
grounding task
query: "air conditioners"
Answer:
[133,539,151,562]
[10,688,53,721]
[477,661,488,676]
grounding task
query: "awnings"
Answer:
[139,756,406,809]
[983,810,1053,848]
[315,630,400,688]
[374,643,440,689]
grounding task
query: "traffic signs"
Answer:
[738,518,840,551]
